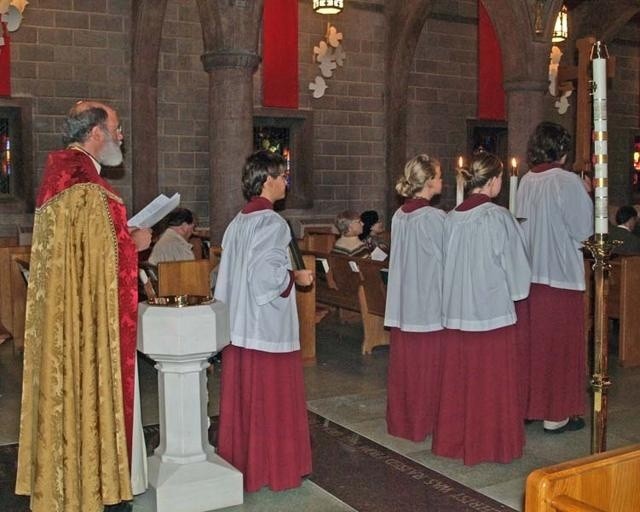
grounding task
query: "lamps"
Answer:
[312,0,343,16]
[551,4,568,43]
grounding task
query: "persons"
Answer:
[14,98,150,512]
[431,151,526,467]
[332,209,387,284]
[361,211,390,255]
[213,150,314,494]
[147,207,197,267]
[516,121,596,431]
[602,203,640,256]
[383,153,446,443]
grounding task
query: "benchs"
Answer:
[0,232,640,369]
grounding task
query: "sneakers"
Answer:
[544,419,585,434]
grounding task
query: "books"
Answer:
[349,261,359,274]
[317,258,328,273]
[285,219,304,272]
[380,269,389,284]
[127,190,182,231]
